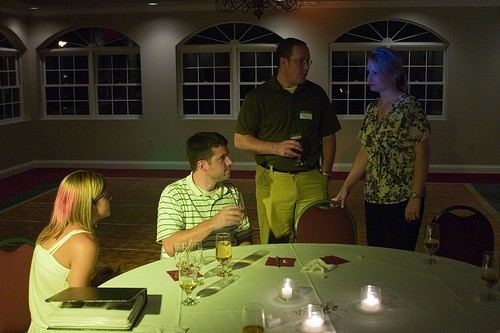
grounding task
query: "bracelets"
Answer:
[319,168,333,176]
[341,186,352,193]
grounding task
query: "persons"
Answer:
[234,37,342,245]
[156,132,254,260]
[26,169,115,333]
[329,47,432,252]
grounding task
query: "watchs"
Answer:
[410,192,423,198]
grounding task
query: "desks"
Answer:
[47,243,500,333]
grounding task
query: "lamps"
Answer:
[215,0,302,21]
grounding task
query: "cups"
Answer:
[242,303,265,333]
[229,193,246,219]
[174,240,204,269]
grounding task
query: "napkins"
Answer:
[266,257,296,267]
[167,270,203,282]
[320,256,350,265]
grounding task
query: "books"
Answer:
[44,287,149,331]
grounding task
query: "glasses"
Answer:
[92,191,112,203]
[283,56,312,66]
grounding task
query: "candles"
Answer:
[302,304,326,333]
[281,278,294,299]
[360,285,381,312]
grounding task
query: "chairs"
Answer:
[0,238,35,333]
[431,205,494,268]
[294,199,358,245]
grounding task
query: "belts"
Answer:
[257,162,320,174]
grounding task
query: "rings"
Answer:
[412,217,415,220]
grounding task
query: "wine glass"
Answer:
[479,252,500,303]
[178,260,198,306]
[216,233,233,277]
[289,133,306,167]
[424,224,441,265]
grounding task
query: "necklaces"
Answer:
[378,95,399,117]
